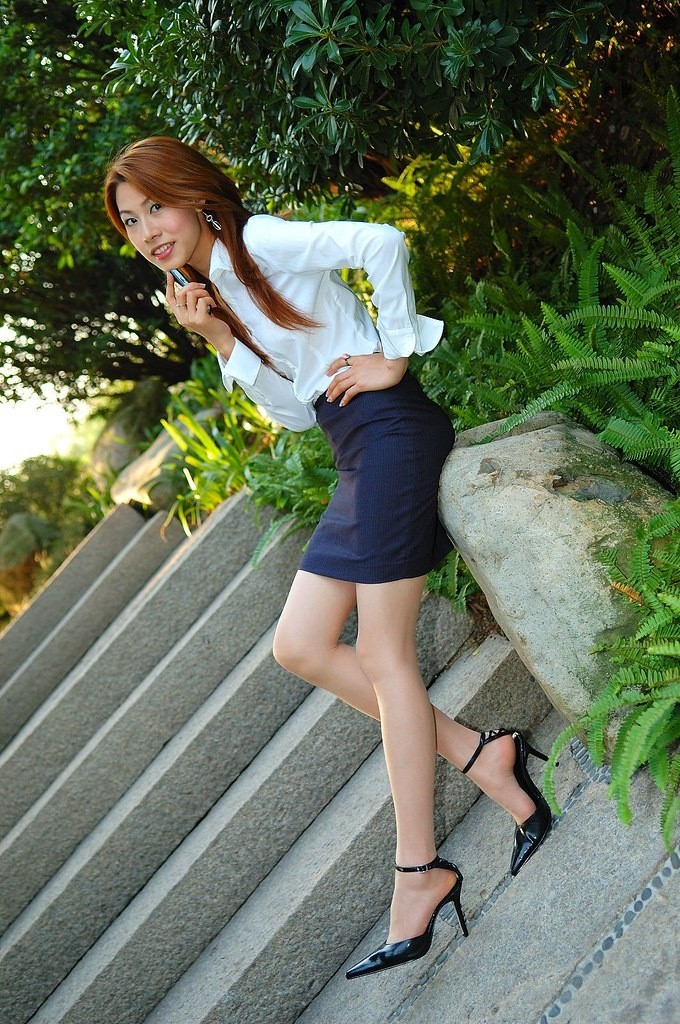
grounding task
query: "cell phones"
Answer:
[171,270,211,315]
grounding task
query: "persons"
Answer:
[106,134,558,978]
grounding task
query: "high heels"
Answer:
[346,855,468,979]
[461,728,551,876]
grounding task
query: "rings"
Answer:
[177,303,185,307]
[342,355,353,366]
